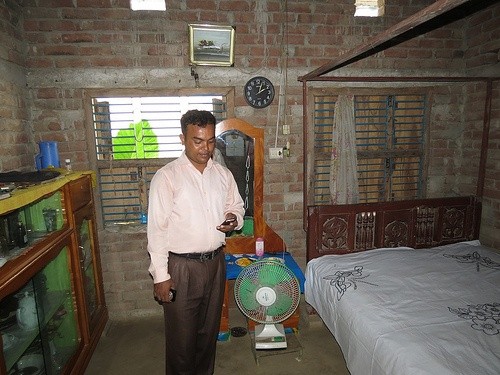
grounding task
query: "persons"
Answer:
[146,110,245,375]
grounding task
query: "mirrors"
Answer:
[215,119,264,253]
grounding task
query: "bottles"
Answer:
[17,219,29,248]
[65,159,71,172]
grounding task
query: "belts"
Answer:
[169,245,225,263]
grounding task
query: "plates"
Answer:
[234,257,256,267]
[264,257,286,264]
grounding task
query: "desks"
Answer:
[218,253,305,340]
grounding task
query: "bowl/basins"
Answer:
[8,354,45,375]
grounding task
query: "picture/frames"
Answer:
[190,24,236,68]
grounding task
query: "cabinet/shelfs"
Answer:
[0,170,108,375]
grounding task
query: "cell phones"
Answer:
[220,218,236,226]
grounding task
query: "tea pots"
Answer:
[16,291,44,331]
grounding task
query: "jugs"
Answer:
[36,140,61,170]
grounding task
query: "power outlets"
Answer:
[269,147,284,160]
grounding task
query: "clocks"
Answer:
[244,77,275,108]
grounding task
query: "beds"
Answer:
[298,0,500,375]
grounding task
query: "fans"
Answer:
[234,262,300,350]
